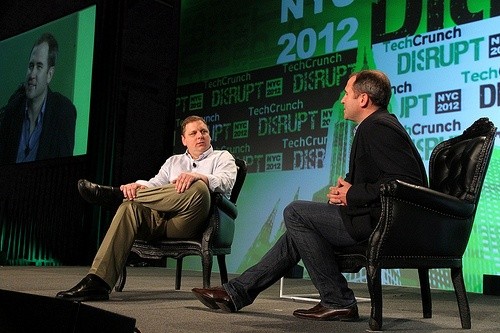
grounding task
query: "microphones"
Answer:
[193,163,196,167]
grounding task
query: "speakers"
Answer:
[0,289,136,333]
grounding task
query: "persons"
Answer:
[0,33,77,165]
[193,70,428,321]
[56,116,238,301]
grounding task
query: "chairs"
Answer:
[115,158,247,292]
[335,117,498,331]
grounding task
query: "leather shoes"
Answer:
[56,275,110,302]
[191,286,236,313]
[77,178,113,206]
[293,301,360,322]
